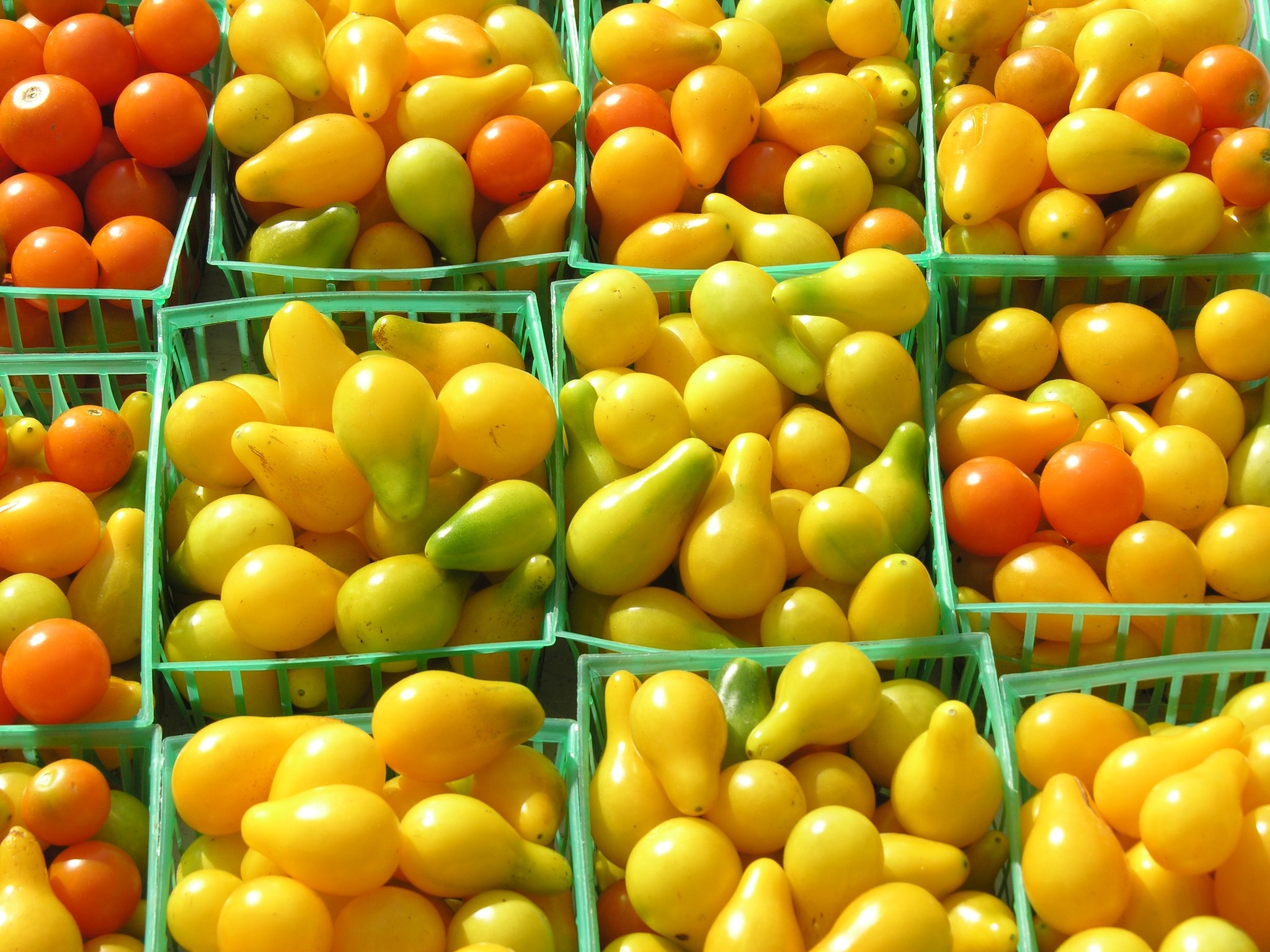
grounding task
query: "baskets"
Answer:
[160,713,595,952]
[0,352,168,781]
[1,723,162,952]
[552,271,960,680]
[206,1,580,339]
[577,632,1032,952]
[915,0,1270,261]
[998,650,1270,952]
[567,0,943,278]
[926,254,1270,725]
[157,291,559,729]
[0,0,228,354]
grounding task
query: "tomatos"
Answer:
[0,0,1270,952]
